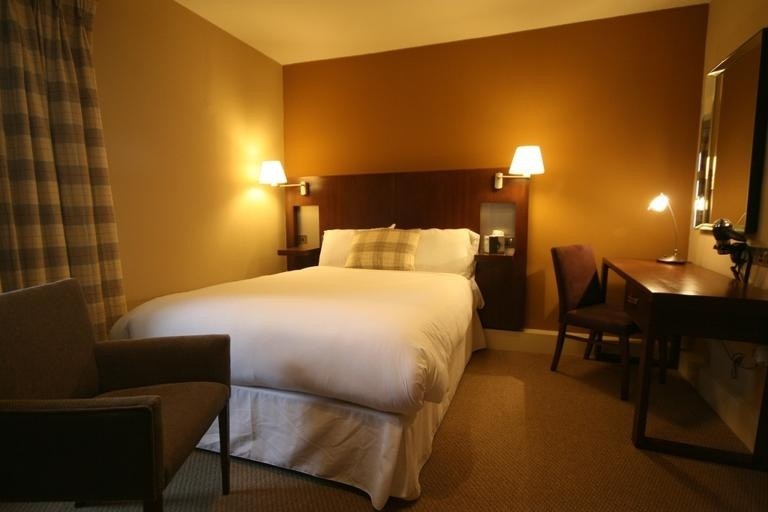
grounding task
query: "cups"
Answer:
[483,235,506,254]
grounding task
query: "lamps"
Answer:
[492,144,546,191]
[647,192,689,265]
[258,160,311,199]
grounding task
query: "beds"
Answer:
[108,225,491,511]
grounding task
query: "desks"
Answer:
[582,256,767,472]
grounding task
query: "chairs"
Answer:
[548,242,644,401]
[2,274,234,512]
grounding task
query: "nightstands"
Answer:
[276,244,320,272]
[472,246,516,265]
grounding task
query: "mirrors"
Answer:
[689,26,766,236]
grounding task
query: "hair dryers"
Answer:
[712,218,745,254]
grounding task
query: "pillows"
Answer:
[342,228,421,271]
[317,222,396,269]
[413,226,480,280]
